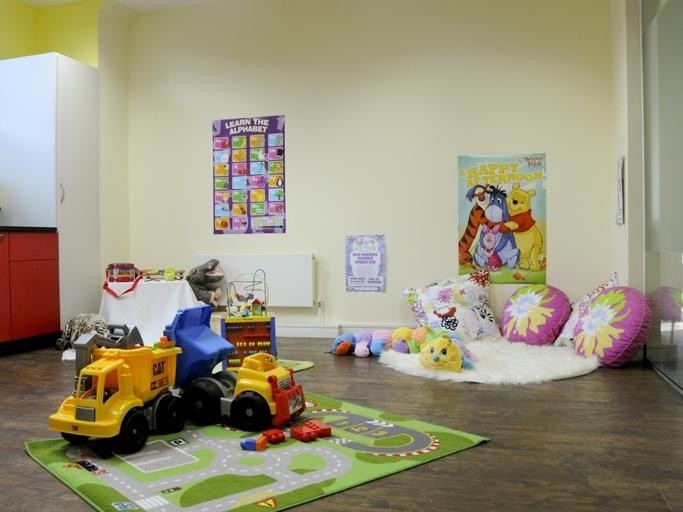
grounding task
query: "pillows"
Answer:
[403,269,652,369]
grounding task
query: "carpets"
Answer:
[21,391,492,512]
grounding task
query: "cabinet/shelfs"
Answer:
[0,52,100,357]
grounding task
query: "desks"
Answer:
[103,280,189,333]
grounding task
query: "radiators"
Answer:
[188,255,315,307]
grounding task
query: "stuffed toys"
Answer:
[185,259,223,312]
[321,325,478,374]
[56,313,110,351]
